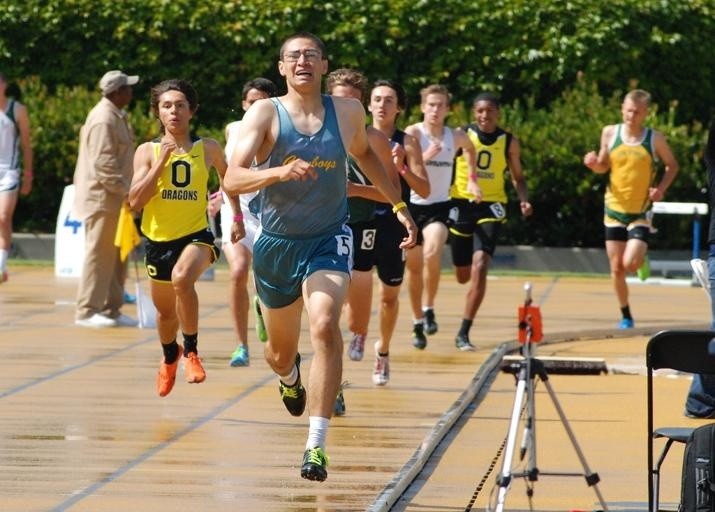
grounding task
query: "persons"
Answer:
[73,70,138,328]
[0,74,33,283]
[128,78,246,397]
[445,95,533,352]
[323,68,482,386]
[584,90,679,332]
[217,77,274,368]
[685,120,715,420]
[224,32,418,483]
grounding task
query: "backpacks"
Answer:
[677,422,715,512]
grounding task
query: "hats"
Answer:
[99,70,140,96]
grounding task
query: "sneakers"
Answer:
[373,340,390,385]
[684,405,715,419]
[301,448,329,481]
[423,308,438,333]
[279,352,307,417]
[637,252,652,281]
[620,318,635,329]
[229,345,251,367]
[349,330,369,361]
[412,319,428,348]
[183,351,206,384]
[74,314,114,328]
[454,333,476,350]
[254,295,267,341]
[333,379,349,418]
[113,313,140,327]
[156,344,185,397]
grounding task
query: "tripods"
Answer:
[484,352,607,512]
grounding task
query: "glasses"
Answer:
[281,51,323,62]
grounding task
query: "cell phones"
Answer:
[518,301,543,343]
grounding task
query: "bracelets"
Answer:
[23,170,32,177]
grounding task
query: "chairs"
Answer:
[646,329,714,511]
